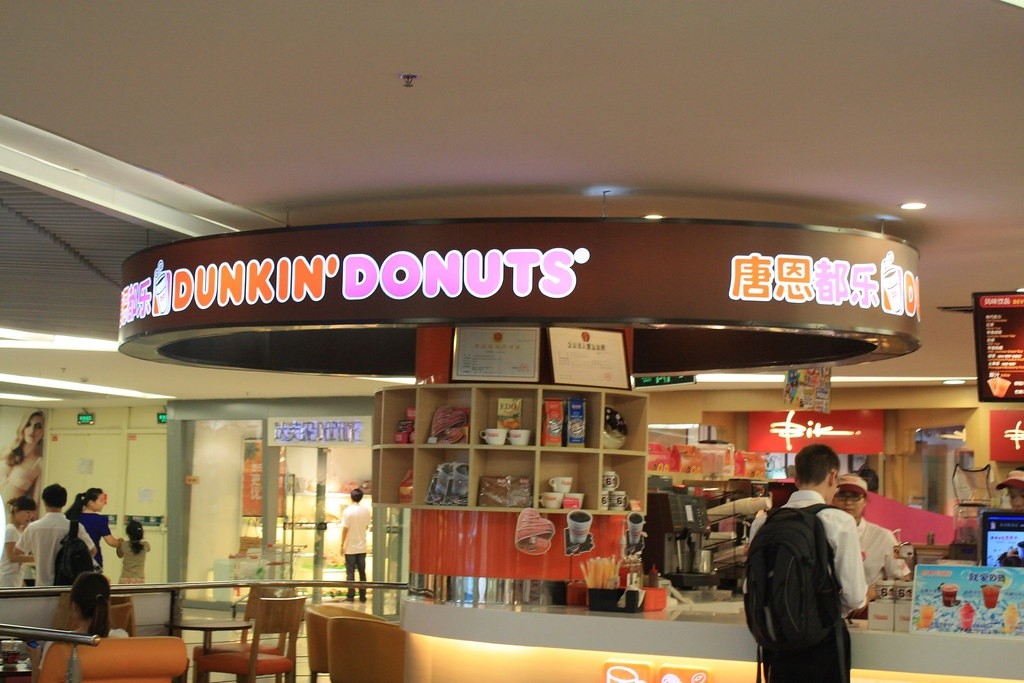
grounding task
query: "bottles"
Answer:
[649,565,658,587]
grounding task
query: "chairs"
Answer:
[40,586,408,683]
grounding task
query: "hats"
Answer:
[835,474,868,496]
[995,470,1024,492]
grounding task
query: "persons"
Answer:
[12,483,98,585]
[995,466,1024,512]
[340,488,371,602]
[0,408,45,526]
[23,572,129,683]
[832,475,912,619]
[116,519,150,584]
[741,444,868,683]
[0,496,36,587]
[65,488,123,571]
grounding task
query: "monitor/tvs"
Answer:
[975,509,1024,568]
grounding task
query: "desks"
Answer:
[159,620,252,682]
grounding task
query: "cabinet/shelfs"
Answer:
[370,381,651,583]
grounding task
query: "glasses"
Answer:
[834,489,867,502]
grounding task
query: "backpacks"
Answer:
[743,503,845,651]
[54,521,94,587]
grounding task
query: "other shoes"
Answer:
[341,597,354,604]
[360,597,366,603]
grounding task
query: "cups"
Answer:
[538,491,563,509]
[507,430,531,445]
[479,428,507,444]
[600,490,609,510]
[563,493,584,508]
[567,509,593,545]
[549,476,573,493]
[626,512,644,545]
[1,641,23,668]
[602,470,619,491]
[610,490,629,510]
[919,586,1019,633]
